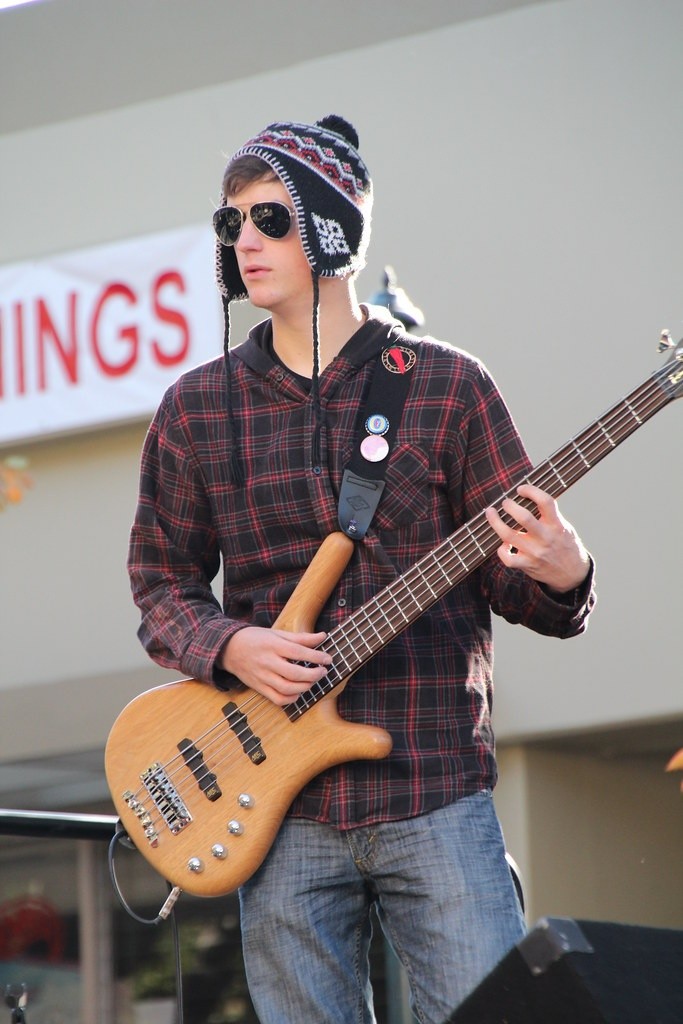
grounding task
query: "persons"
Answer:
[126,112,599,1024]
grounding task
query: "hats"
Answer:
[215,114,394,488]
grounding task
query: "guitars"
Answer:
[105,328,680,899]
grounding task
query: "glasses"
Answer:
[213,201,292,247]
